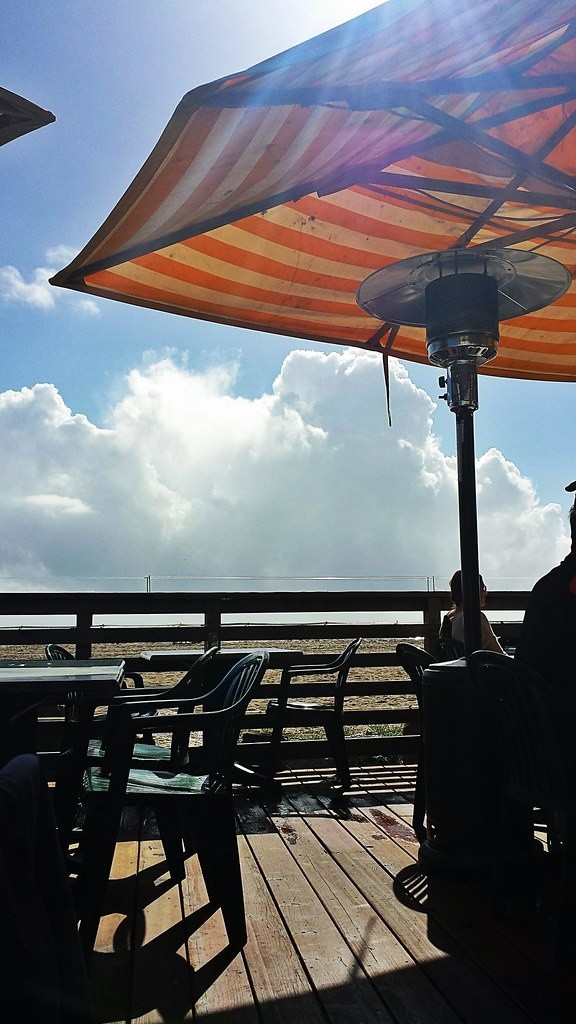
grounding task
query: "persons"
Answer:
[437,566,509,658]
[518,494,576,685]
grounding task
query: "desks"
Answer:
[0,659,126,769]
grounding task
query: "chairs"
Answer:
[52,637,576,963]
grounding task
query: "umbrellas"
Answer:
[0,87,56,150]
[44,0,576,650]
